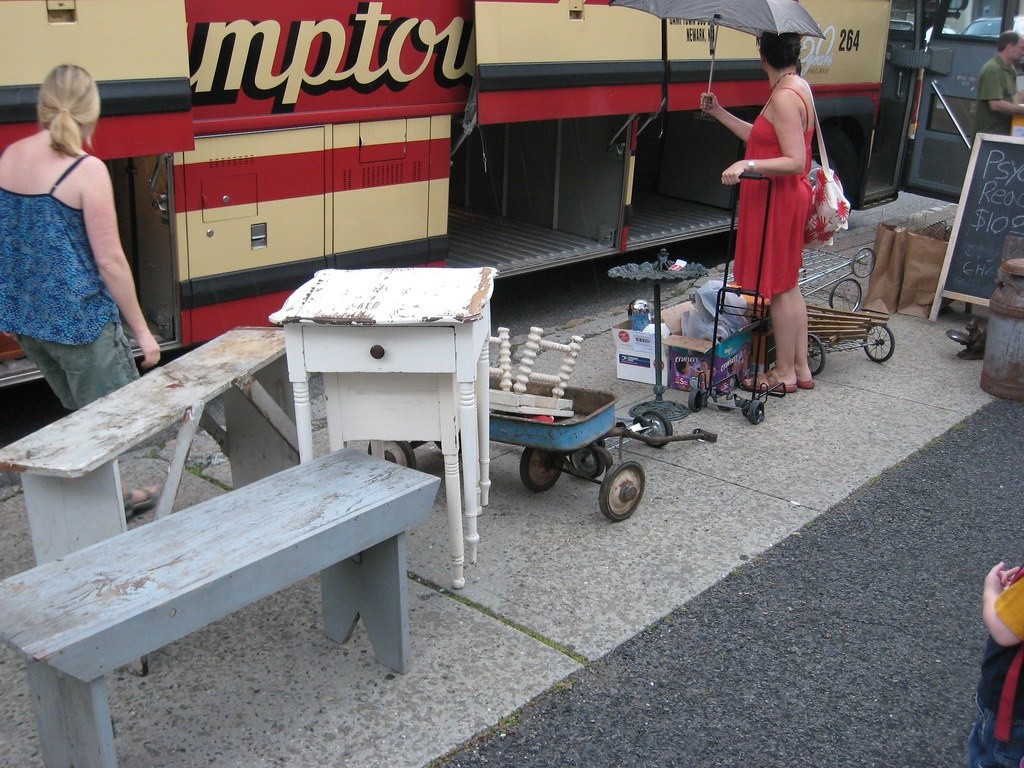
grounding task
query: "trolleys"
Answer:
[486,377,717,525]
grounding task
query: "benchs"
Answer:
[0,325,302,676]
[0,448,442,768]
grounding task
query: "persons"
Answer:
[699,32,814,391]
[967,560,1024,768]
[0,66,165,520]
[973,31,1024,137]
[737,348,751,380]
[676,358,716,388]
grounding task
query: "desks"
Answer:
[268,267,500,587]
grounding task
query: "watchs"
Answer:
[747,159,755,172]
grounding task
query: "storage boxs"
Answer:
[612,282,777,395]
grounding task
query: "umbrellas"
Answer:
[608,0,826,111]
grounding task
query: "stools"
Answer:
[489,328,582,418]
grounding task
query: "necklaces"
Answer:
[769,71,799,93]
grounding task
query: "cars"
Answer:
[959,14,1024,39]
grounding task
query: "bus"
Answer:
[0,0,1004,395]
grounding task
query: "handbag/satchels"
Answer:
[863,210,954,317]
[804,167,850,250]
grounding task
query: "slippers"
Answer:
[796,381,815,389]
[742,371,797,393]
